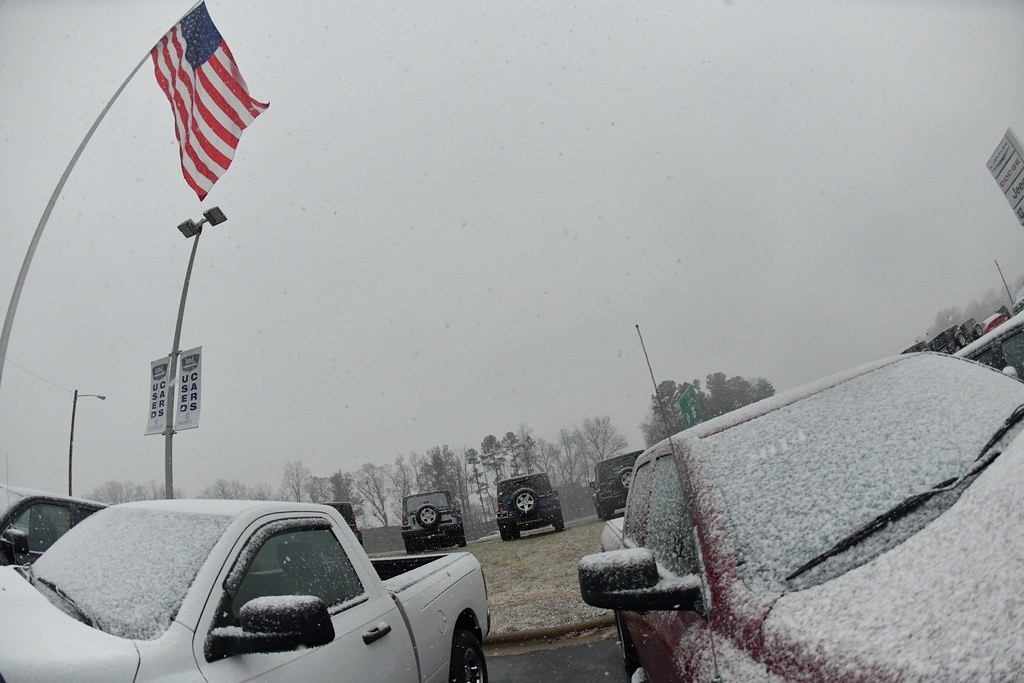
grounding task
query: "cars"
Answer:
[953,308,1024,383]
[979,313,1008,335]
[1012,287,1024,314]
[578,352,1024,683]
[0,485,112,567]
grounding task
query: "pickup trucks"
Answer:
[0,496,492,683]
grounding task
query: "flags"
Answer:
[149,1,271,201]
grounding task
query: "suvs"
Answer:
[959,317,984,344]
[272,503,363,556]
[588,449,657,522]
[900,341,932,354]
[924,324,969,355]
[401,490,467,554]
[496,472,566,541]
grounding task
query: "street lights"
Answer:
[68,389,106,497]
[163,206,228,497]
